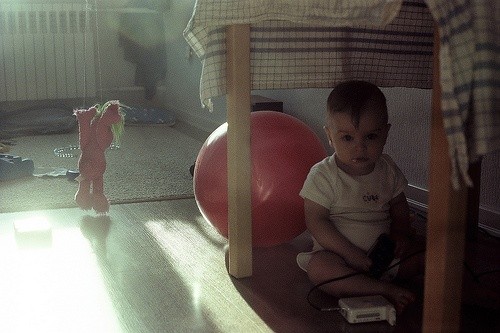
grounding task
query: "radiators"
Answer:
[0,4,97,101]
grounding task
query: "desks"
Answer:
[182,1,500,333]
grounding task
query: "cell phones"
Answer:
[366,232,396,279]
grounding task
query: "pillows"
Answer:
[123,106,177,128]
[1,108,77,136]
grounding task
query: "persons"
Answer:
[296,81,426,317]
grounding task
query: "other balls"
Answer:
[193,111,327,248]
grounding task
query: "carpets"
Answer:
[0,125,203,213]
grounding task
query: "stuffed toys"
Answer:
[72,99,125,214]
